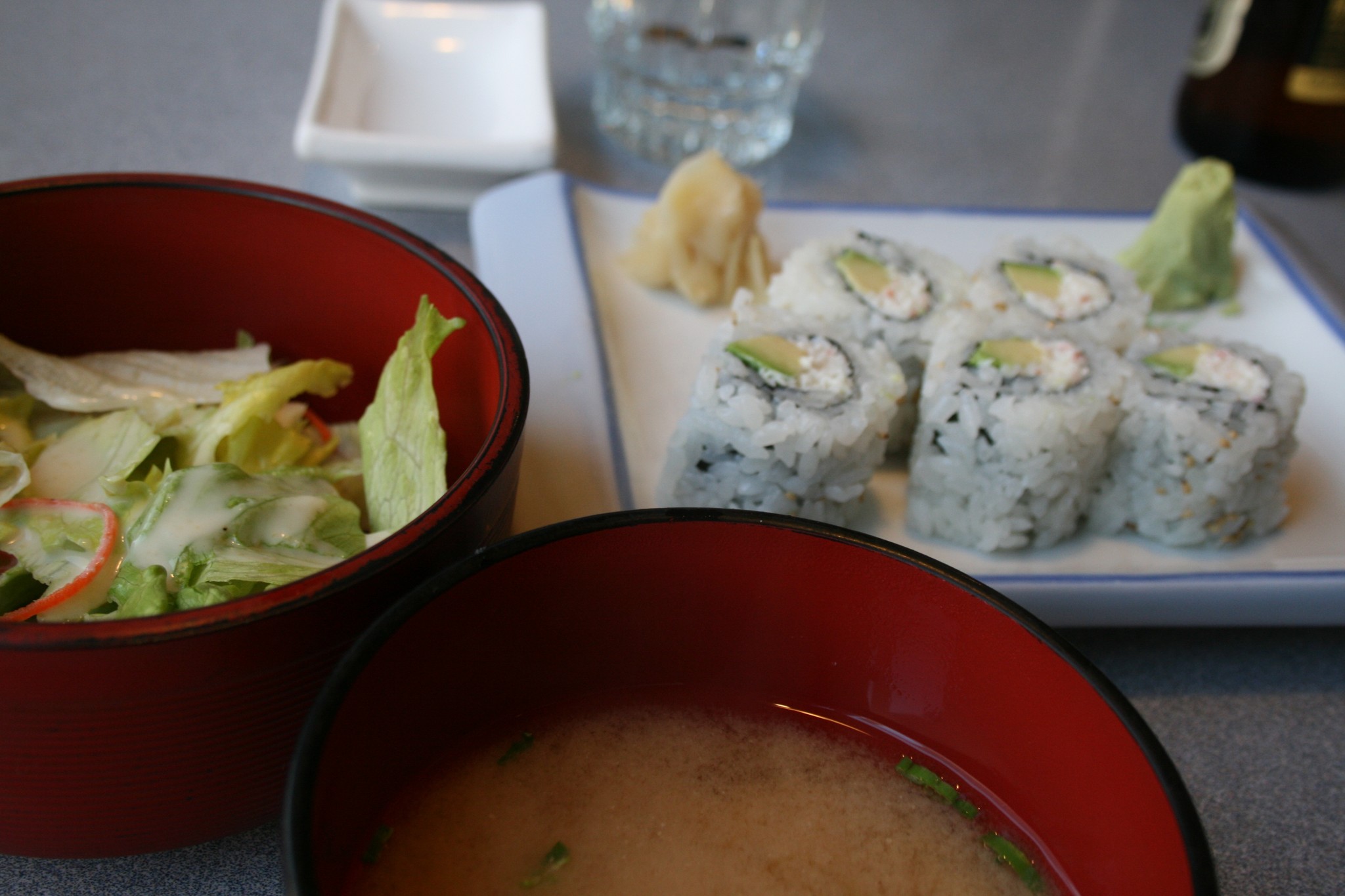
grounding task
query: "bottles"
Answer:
[1180,0,1344,189]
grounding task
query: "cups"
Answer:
[582,1,822,169]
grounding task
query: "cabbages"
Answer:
[1,296,466,620]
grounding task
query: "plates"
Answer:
[467,170,1345,635]
[292,1,559,215]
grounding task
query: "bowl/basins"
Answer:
[0,171,530,861]
[280,508,1225,896]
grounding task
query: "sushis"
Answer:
[649,226,1305,555]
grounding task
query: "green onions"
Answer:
[496,732,1043,894]
